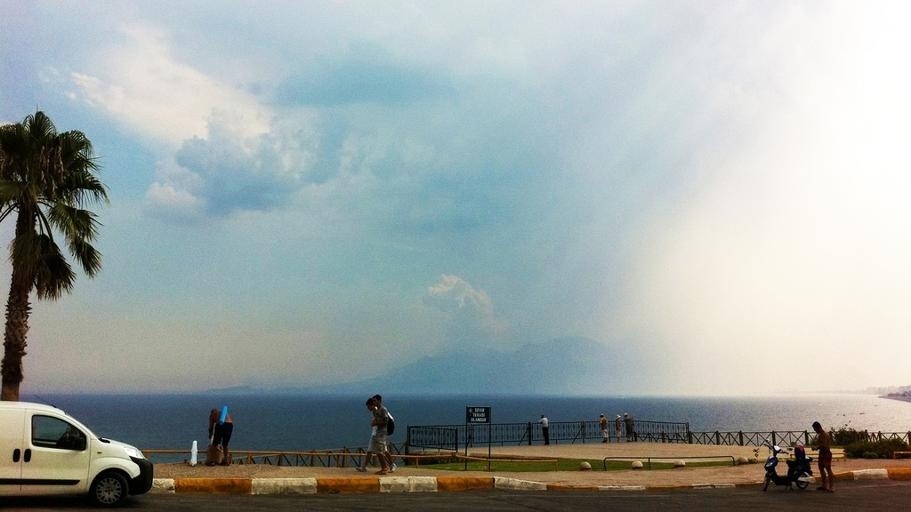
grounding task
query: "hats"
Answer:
[599,412,628,419]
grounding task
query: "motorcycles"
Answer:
[763,439,814,491]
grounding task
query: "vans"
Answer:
[0,399,156,503]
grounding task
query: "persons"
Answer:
[538,414,550,445]
[599,413,609,443]
[356,398,398,472]
[615,414,622,443]
[623,413,632,442]
[370,394,388,475]
[206,407,233,466]
[812,421,835,493]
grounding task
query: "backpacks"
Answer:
[386,415,395,435]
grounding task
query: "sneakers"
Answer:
[388,463,397,473]
[375,469,387,476]
[815,486,836,493]
[355,466,367,472]
[602,440,632,443]
